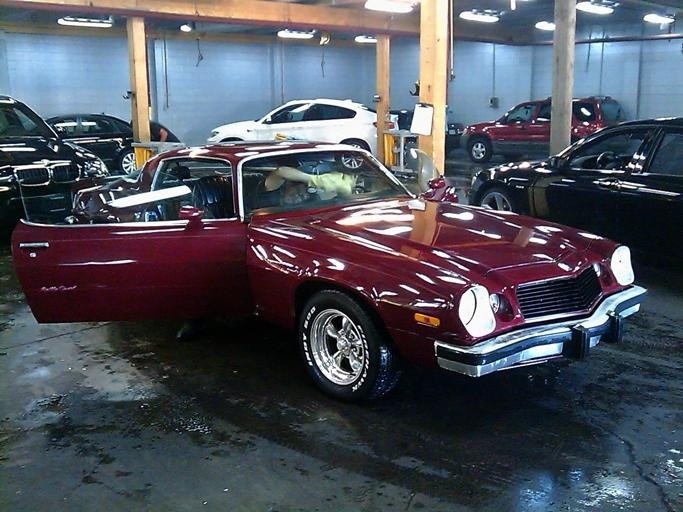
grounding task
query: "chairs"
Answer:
[192,175,248,219]
[161,167,196,220]
[243,173,264,209]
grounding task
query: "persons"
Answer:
[249,165,356,213]
[149,122,180,144]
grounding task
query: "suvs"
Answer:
[461,96,628,162]
[206,97,398,170]
[0,94,110,225]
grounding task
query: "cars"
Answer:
[26,113,137,177]
[11,137,647,406]
[469,116,682,265]
[389,109,464,154]
